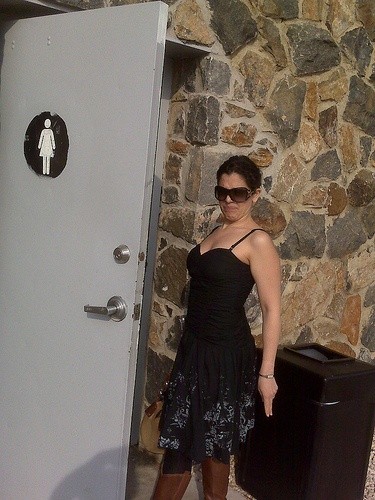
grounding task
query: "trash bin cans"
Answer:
[237,341,375,500]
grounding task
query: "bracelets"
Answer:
[259,371,275,379]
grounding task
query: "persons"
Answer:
[147,156,284,500]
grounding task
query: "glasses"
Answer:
[215,185,254,202]
